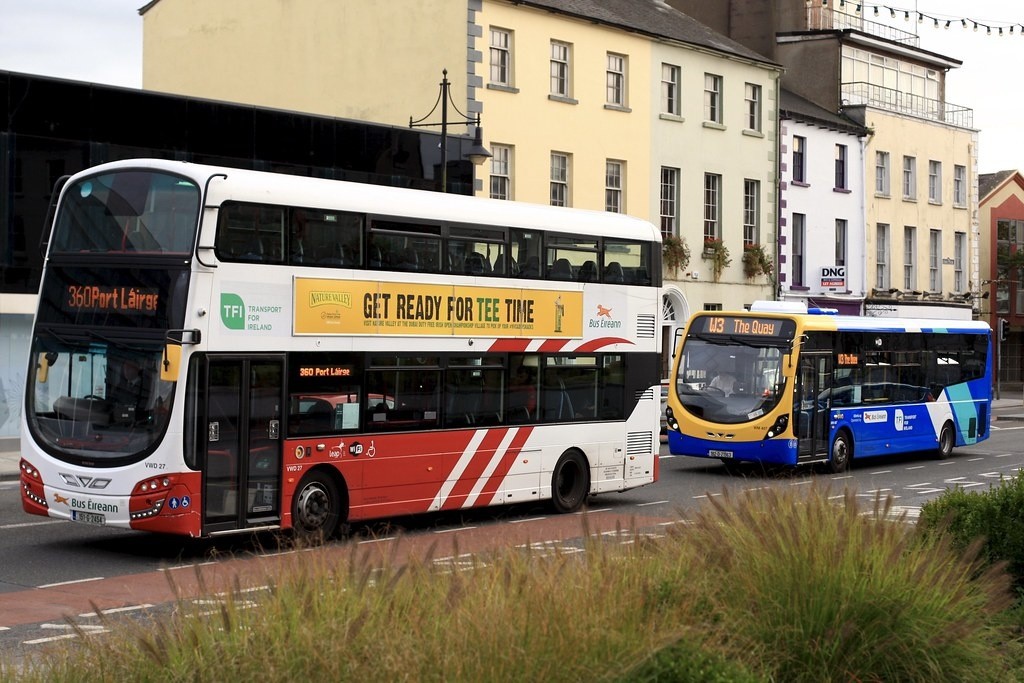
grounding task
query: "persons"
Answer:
[104,358,152,409]
[508,365,537,412]
[353,220,407,267]
[706,363,738,398]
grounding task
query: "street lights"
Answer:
[403,70,493,272]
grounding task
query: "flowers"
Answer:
[744,243,777,286]
[705,236,734,282]
[663,234,691,274]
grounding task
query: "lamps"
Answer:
[872,287,990,301]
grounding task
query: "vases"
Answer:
[705,247,715,254]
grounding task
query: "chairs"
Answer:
[850,363,983,401]
[297,377,575,437]
[106,209,624,284]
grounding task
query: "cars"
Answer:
[660,382,696,436]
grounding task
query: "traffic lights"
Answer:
[1001,319,1011,341]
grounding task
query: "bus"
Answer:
[19,157,664,550]
[665,300,993,474]
